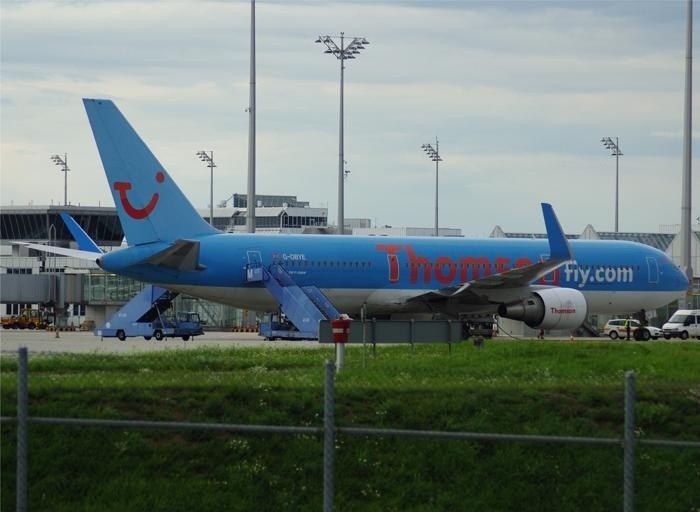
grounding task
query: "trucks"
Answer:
[1,308,40,330]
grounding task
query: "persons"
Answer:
[624,315,631,341]
[462,319,471,340]
[537,329,545,340]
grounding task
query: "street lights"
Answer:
[50,151,71,206]
[421,136,444,236]
[599,136,624,232]
[314,31,370,235]
[196,150,216,223]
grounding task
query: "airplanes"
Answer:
[81,97,690,338]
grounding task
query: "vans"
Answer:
[662,309,700,340]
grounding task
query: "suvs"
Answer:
[604,319,663,341]
